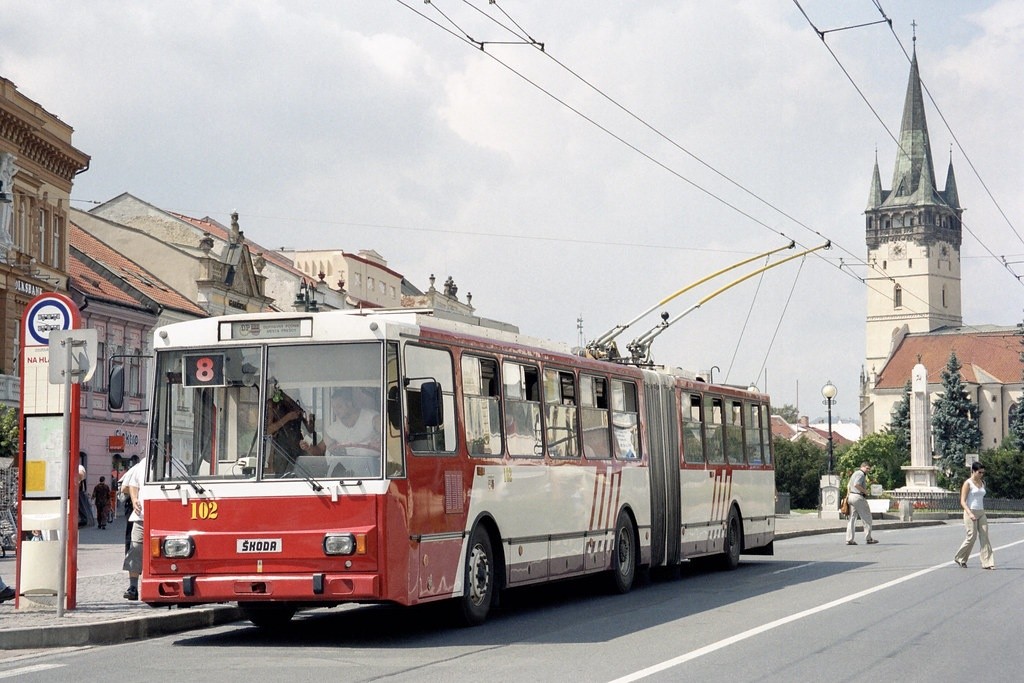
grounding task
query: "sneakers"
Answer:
[124,586,138,600]
[0,586,25,605]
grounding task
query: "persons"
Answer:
[91,476,110,530]
[955,462,996,570]
[267,388,314,478]
[117,434,188,600]
[0,576,16,603]
[299,390,381,457]
[845,462,879,545]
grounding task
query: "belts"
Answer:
[851,492,862,495]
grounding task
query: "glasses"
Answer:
[978,471,985,474]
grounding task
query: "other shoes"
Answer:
[846,541,858,545]
[98,525,100,528]
[867,538,878,543]
[955,560,967,568]
[989,566,996,570]
[102,526,105,529]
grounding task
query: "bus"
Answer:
[106,305,776,630]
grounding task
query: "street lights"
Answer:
[822,381,837,475]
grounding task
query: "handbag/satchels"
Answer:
[842,497,848,515]
